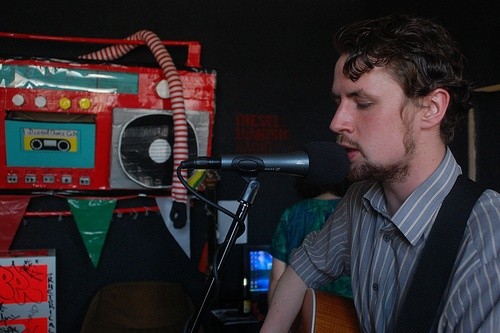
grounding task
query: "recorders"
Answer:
[0,29,216,198]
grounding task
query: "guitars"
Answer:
[290,288,359,333]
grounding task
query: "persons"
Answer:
[265,136,361,313]
[256,20,500,333]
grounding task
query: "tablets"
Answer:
[246,275,271,295]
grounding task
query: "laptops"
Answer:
[245,245,274,274]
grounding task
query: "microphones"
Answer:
[181,147,334,179]
